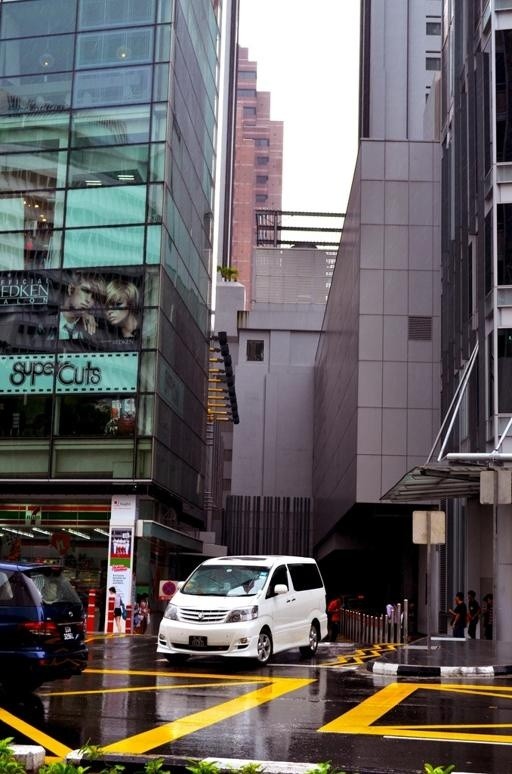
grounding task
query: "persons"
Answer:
[56,272,141,341]
[134,592,152,634]
[353,596,379,616]
[328,596,344,642]
[448,590,493,640]
[386,601,398,643]
[227,573,270,596]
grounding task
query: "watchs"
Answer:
[108,587,122,632]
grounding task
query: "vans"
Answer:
[156,555,329,667]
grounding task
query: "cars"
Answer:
[0,562,89,692]
